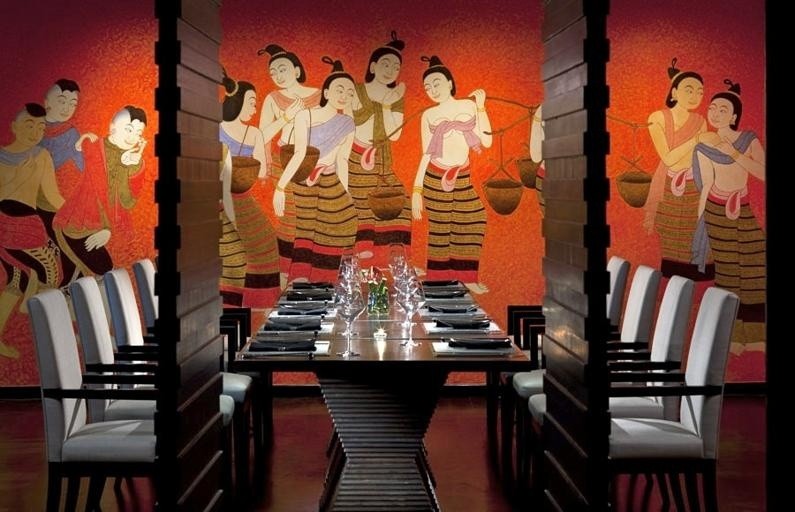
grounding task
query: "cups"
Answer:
[388,244,425,346]
[334,248,366,356]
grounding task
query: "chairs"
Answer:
[526,275,694,512]
[106,268,253,503]
[499,256,631,480]
[133,258,267,467]
[608,287,739,512]
[28,288,158,511]
[511,264,662,488]
[69,276,235,512]
[222,307,273,435]
[486,305,542,475]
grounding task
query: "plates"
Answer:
[278,307,327,315]
[249,339,316,351]
[449,337,511,351]
[431,303,478,313]
[264,319,321,331]
[433,317,490,329]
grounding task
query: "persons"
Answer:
[690,78,767,356]
[530,103,547,220]
[256,41,324,289]
[40,77,100,176]
[0,101,67,361]
[411,53,493,296]
[639,55,715,281]
[273,54,356,290]
[217,75,279,318]
[352,27,426,278]
[50,105,148,323]
